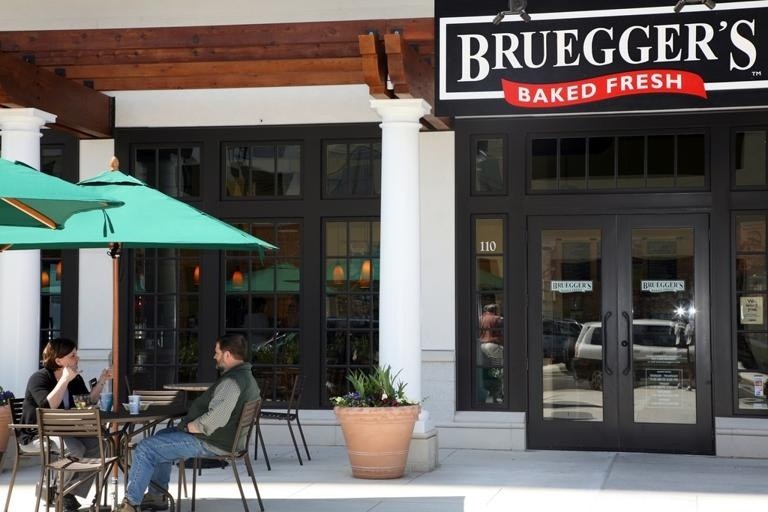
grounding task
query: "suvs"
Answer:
[572,320,696,391]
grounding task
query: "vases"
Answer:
[0,405,13,465]
[333,404,422,480]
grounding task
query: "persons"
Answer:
[673,308,695,392]
[479,302,505,401]
[118,333,262,511]
[17,338,112,512]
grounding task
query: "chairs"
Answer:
[4,398,62,512]
[254,375,311,471]
[177,399,264,512]
[129,390,190,498]
[35,407,118,512]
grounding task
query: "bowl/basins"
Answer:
[123,403,149,412]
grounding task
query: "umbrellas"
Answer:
[0,155,283,511]
[0,156,125,238]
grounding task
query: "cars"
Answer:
[252,317,505,396]
[539,319,581,363]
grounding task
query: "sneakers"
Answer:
[140,492,168,510]
[112,497,140,512]
[55,492,79,512]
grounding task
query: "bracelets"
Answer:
[97,378,107,390]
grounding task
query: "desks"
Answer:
[164,383,229,469]
[69,411,170,512]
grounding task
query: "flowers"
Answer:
[0,386,15,406]
[329,365,408,407]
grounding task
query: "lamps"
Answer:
[232,252,244,289]
[493,0,531,26]
[334,222,344,285]
[673,0,716,13]
[56,261,61,281]
[358,259,371,289]
[41,271,49,288]
[193,266,200,285]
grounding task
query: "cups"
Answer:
[128,395,142,416]
[72,395,90,409]
[100,392,113,412]
